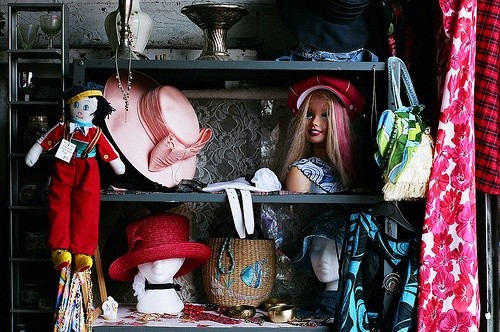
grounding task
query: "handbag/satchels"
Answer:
[375,56,435,201]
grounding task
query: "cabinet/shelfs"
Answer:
[73,59,399,332]
[6,4,71,332]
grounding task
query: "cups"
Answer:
[16,24,39,50]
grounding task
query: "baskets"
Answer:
[202,236,276,309]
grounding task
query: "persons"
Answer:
[109,212,212,314]
[294,210,380,321]
[280,74,367,194]
[24,81,126,272]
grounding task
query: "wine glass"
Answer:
[40,15,61,50]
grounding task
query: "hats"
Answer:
[102,68,212,188]
[108,212,211,280]
[293,210,348,262]
[288,74,365,120]
[274,0,370,52]
